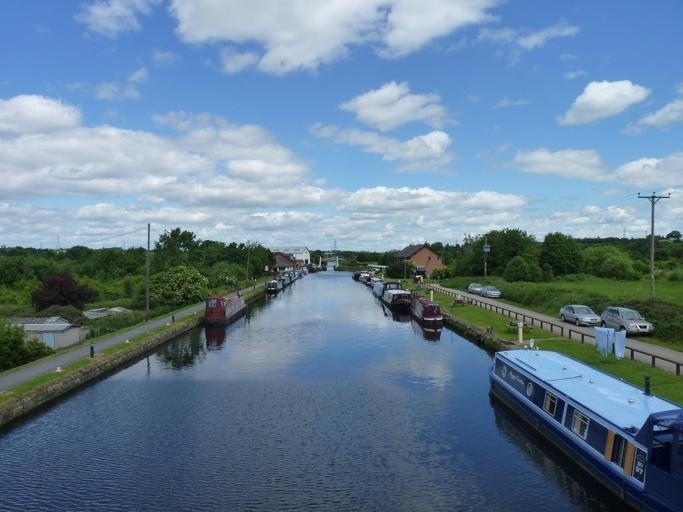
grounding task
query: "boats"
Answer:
[203,311,246,351]
[380,302,411,323]
[380,289,414,309]
[352,270,403,296]
[487,398,640,512]
[266,264,328,293]
[489,349,682,511]
[205,294,248,324]
[408,296,443,333]
[409,319,440,342]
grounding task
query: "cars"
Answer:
[467,283,484,294]
[559,304,601,326]
[480,286,501,298]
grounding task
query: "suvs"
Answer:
[601,306,655,336]
